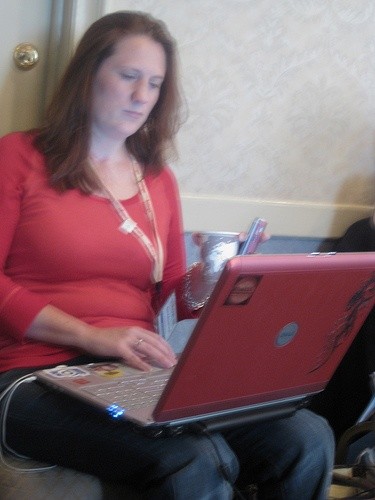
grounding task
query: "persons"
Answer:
[0,10,335,500]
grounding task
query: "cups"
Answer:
[200,234,238,284]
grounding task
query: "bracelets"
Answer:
[185,262,211,311]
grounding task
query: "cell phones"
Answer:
[239,219,267,256]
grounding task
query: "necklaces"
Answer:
[88,155,164,293]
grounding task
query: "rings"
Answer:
[135,339,143,345]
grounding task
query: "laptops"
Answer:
[31,251,375,434]
